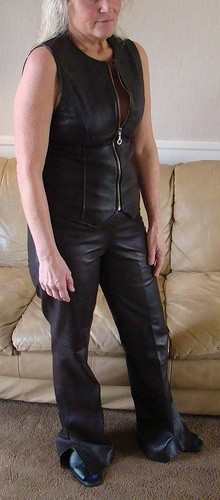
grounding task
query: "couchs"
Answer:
[0,155,220,415]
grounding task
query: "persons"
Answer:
[13,0,204,487]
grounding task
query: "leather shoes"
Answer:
[69,448,103,487]
